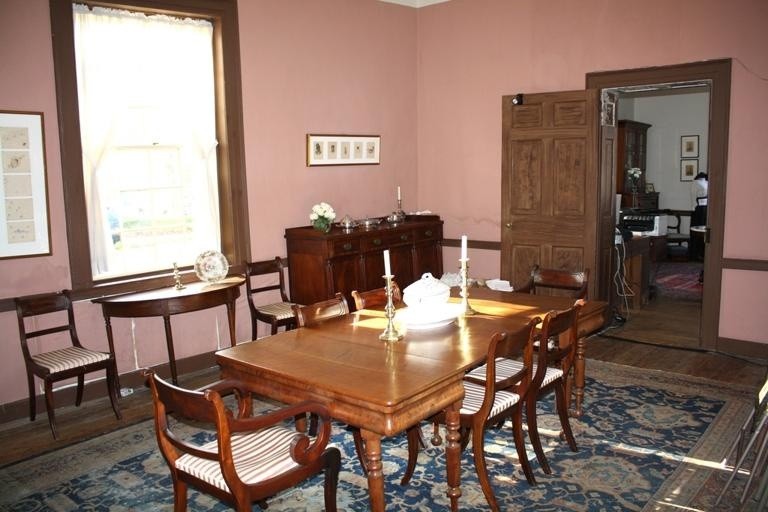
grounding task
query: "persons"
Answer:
[689,170,709,265]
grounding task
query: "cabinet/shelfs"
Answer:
[619,236,649,310]
[281,210,444,329]
[617,119,660,209]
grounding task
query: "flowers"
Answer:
[309,201,336,232]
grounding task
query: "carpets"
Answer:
[0,354,759,512]
[655,275,702,292]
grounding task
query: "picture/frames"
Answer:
[306,133,381,167]
[679,159,700,182]
[1,108,54,263]
[680,135,700,158]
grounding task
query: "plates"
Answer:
[395,304,468,330]
[194,250,228,282]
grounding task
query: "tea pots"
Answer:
[387,213,405,223]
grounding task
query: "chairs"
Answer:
[11,288,123,444]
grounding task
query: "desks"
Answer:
[91,275,247,398]
